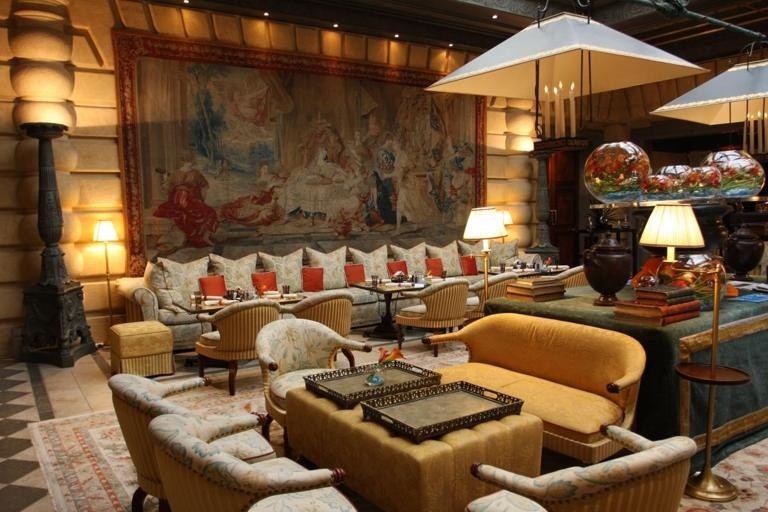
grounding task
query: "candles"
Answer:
[542,84,575,138]
[741,112,768,153]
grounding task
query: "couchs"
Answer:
[114,229,569,352]
[422,312,646,467]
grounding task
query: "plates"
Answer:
[514,269,534,272]
[261,288,299,300]
[204,296,223,305]
[549,265,569,269]
[365,278,443,288]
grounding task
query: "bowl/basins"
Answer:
[505,267,513,272]
[491,266,500,272]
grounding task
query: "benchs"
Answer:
[276,388,543,510]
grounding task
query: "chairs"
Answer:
[393,269,519,356]
[253,320,373,440]
[460,424,695,512]
[108,371,357,512]
[188,289,356,397]
[551,266,588,289]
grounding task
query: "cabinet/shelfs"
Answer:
[530,141,587,264]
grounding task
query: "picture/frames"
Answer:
[107,28,486,278]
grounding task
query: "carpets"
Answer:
[665,439,768,512]
[29,327,757,511]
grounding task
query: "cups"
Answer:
[372,275,378,288]
[535,263,540,272]
[441,271,446,278]
[195,295,202,307]
[517,265,521,269]
[500,258,506,274]
[398,271,423,283]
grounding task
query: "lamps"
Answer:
[639,203,706,270]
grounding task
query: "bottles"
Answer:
[227,287,254,301]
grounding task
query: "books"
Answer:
[505,281,565,294]
[616,280,703,324]
[504,292,567,303]
[518,275,558,286]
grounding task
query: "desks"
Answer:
[480,278,767,472]
